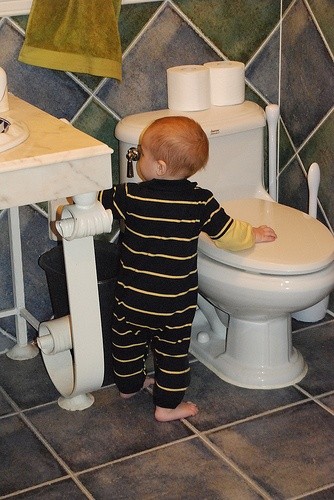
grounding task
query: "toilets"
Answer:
[114,98,334,391]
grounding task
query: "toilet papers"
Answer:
[165,63,212,113]
[203,59,248,107]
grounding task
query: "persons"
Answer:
[65,116,277,422]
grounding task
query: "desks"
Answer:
[0,91,113,411]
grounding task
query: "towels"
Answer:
[16,0,123,86]
